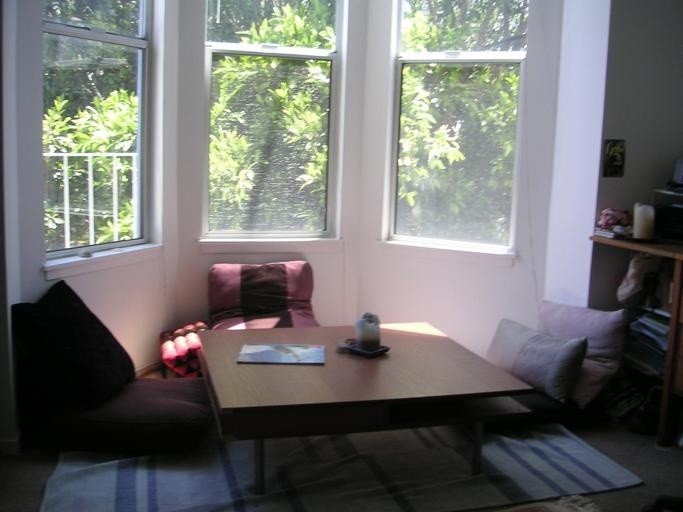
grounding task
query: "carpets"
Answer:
[40,423,644,512]
[498,494,605,511]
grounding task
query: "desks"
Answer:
[194,321,534,497]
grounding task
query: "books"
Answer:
[237,343,325,366]
[594,228,622,240]
[625,282,675,377]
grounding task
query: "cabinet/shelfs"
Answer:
[589,234,683,450]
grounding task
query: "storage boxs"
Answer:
[651,187,683,245]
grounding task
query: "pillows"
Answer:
[11,278,135,411]
[19,374,219,457]
[534,298,629,409]
[485,318,588,404]
[207,260,322,330]
